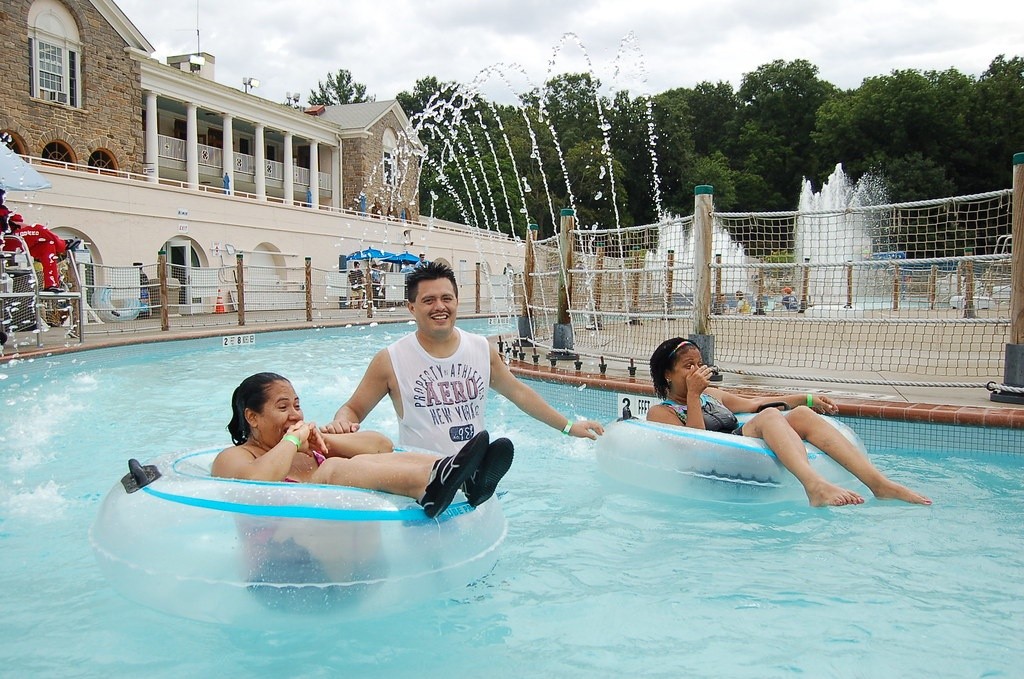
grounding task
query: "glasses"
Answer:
[421,257,425,258]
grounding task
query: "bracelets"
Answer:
[280,435,301,449]
[562,420,573,435]
[807,393,813,408]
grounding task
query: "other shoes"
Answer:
[45,286,65,292]
[63,237,81,251]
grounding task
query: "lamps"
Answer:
[206,113,215,117]
[244,78,260,93]
[266,131,274,135]
[287,92,300,107]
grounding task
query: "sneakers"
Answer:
[419,430,514,520]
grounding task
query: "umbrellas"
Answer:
[306,186,313,207]
[223,172,231,194]
[346,247,420,264]
[361,194,367,218]
[0,143,52,193]
[401,207,406,220]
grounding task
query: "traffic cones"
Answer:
[212,289,228,314]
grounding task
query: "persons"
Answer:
[713,287,796,317]
[0,189,81,291]
[320,263,605,460]
[348,261,381,307]
[400,254,430,274]
[212,373,514,611]
[649,338,932,509]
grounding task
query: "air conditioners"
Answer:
[49,90,68,105]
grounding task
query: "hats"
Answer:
[0,205,27,226]
[372,263,378,268]
[784,287,791,294]
